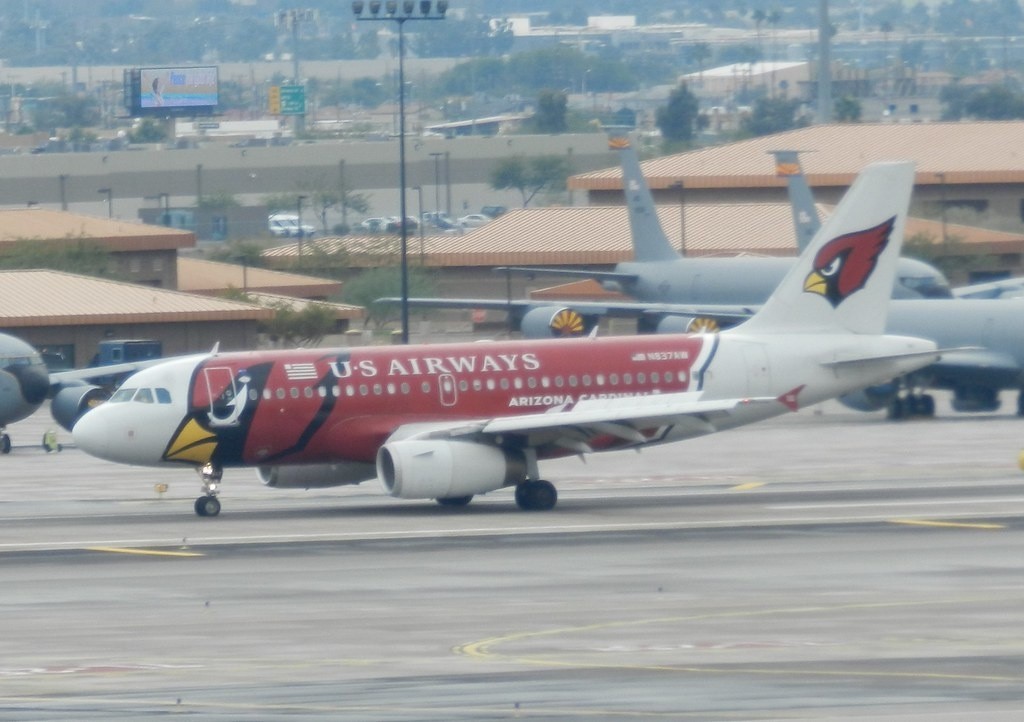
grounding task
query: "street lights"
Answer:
[351,0,449,343]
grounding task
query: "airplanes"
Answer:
[373,117,1023,423]
[70,160,982,524]
[0,331,222,454]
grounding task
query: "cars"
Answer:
[269,204,511,237]
